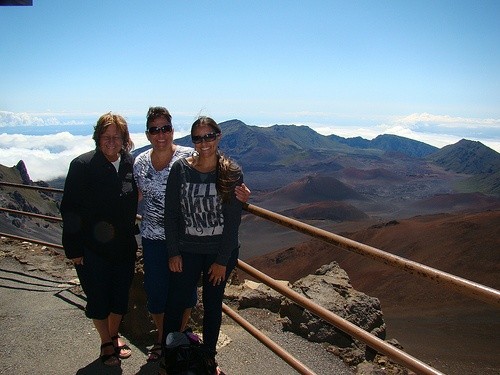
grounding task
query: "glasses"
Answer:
[192,132,220,144]
[149,125,172,135]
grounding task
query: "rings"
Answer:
[218,278,221,281]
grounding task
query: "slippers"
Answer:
[207,359,217,375]
[157,359,169,375]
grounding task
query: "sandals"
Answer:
[111,334,132,358]
[151,342,162,361]
[100,341,121,367]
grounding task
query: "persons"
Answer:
[132,106,251,362]
[61,112,141,367]
[164,116,245,375]
